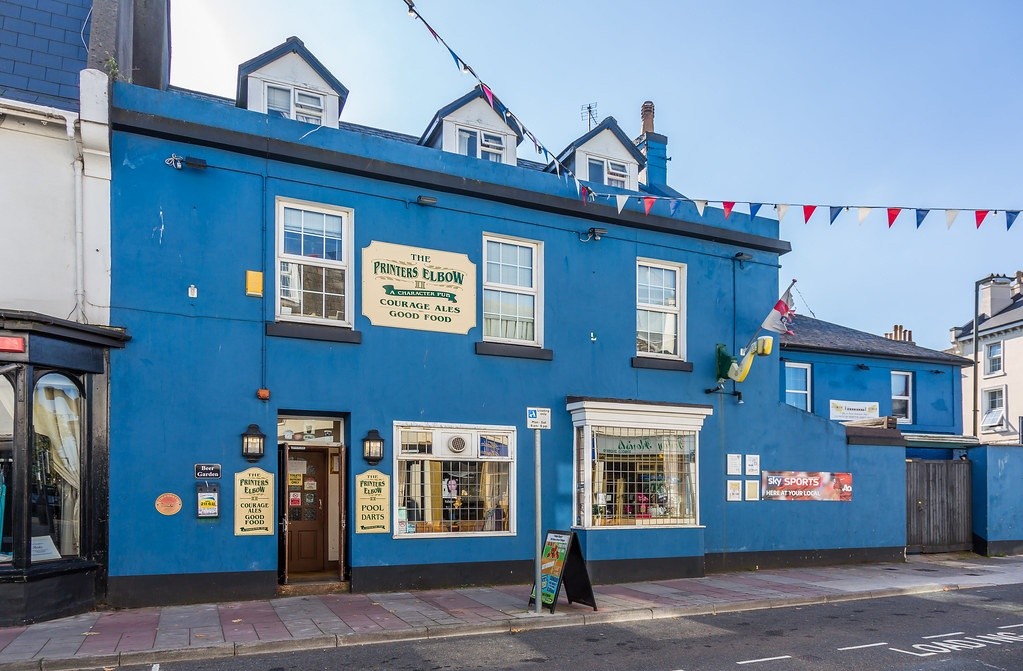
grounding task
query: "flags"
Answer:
[762,289,796,336]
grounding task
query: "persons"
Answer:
[447,478,457,496]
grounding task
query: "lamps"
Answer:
[361,429,384,465]
[240,424,266,463]
